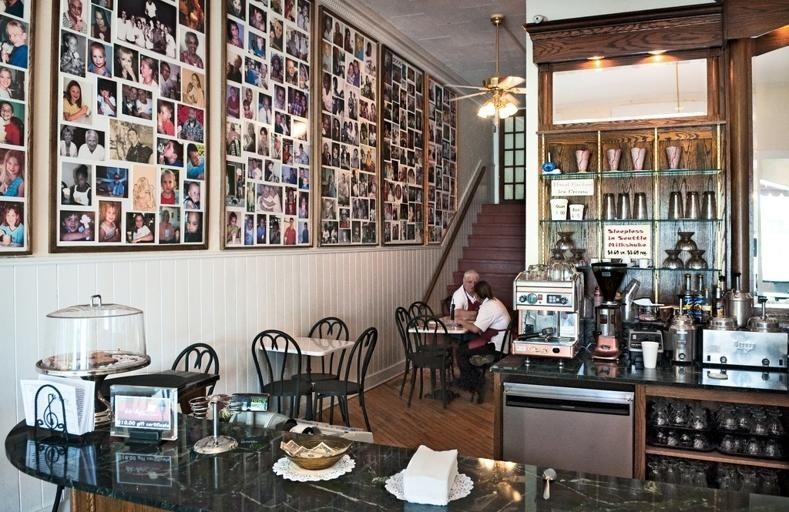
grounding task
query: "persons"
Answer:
[429,80,454,241]
[60,2,204,244]
[2,2,29,246]
[450,268,481,392]
[225,1,309,245]
[321,13,377,243]
[454,280,515,404]
[384,50,425,242]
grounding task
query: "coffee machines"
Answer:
[512,271,585,368]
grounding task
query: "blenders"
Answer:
[591,262,627,362]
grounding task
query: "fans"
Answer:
[444,13,526,102]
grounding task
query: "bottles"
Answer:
[681,273,724,325]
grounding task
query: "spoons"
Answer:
[542,468,557,499]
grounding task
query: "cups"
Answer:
[654,429,782,457]
[666,147,681,168]
[631,148,646,170]
[602,192,648,221]
[607,149,622,170]
[642,342,659,369]
[575,149,589,172]
[667,191,718,221]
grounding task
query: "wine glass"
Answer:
[647,396,784,436]
[548,232,588,267]
[662,232,709,271]
[647,454,781,496]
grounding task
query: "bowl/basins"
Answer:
[280,440,352,470]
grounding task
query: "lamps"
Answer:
[478,99,517,118]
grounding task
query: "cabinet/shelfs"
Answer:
[536,123,725,307]
[646,392,788,497]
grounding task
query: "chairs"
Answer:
[406,316,452,409]
[313,327,378,433]
[395,307,443,397]
[168,343,220,402]
[251,329,313,421]
[292,317,350,424]
[408,302,458,379]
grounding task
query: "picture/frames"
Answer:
[427,74,457,245]
[48,0,211,253]
[222,1,315,248]
[380,46,426,245]
[317,5,378,246]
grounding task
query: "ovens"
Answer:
[501,378,634,480]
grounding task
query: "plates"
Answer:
[385,469,473,506]
[274,458,355,482]
